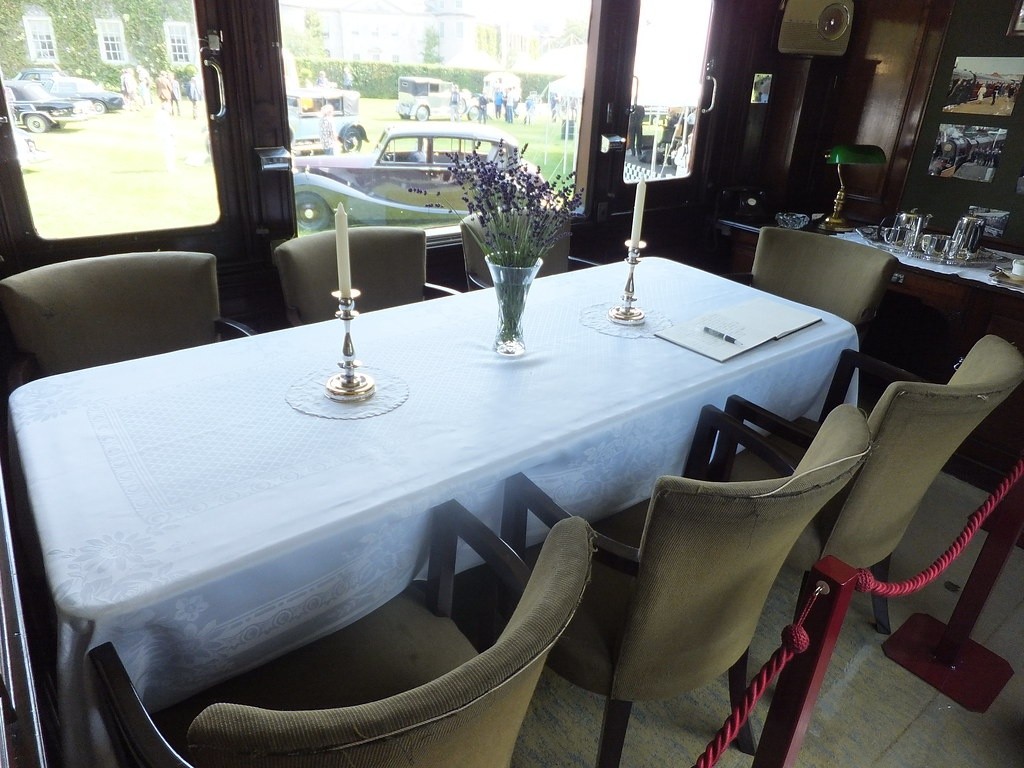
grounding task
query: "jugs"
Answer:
[889,208,933,246]
[953,215,986,251]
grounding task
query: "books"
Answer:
[653,298,823,363]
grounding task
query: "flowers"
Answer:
[443,138,586,339]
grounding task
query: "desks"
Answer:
[713,218,1023,459]
[6,256,858,767]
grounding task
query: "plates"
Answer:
[993,270,1024,286]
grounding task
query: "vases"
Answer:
[485,251,544,356]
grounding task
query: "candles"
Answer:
[334,202,351,296]
[632,174,646,247]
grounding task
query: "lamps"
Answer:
[819,144,886,233]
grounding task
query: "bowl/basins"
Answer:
[776,212,807,229]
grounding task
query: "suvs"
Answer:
[282,48,368,154]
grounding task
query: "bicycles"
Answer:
[467,105,493,121]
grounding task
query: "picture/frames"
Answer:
[1003,0,1024,36]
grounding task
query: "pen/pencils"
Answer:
[704,327,743,346]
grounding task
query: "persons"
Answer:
[314,104,336,155]
[120,65,154,113]
[154,70,182,117]
[948,78,1023,106]
[188,75,202,121]
[548,91,578,124]
[478,86,538,126]
[342,63,353,90]
[314,70,330,90]
[448,84,462,122]
[969,147,1001,168]
[657,108,697,147]
[629,106,645,157]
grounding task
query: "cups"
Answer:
[900,230,957,259]
[1013,259,1024,276]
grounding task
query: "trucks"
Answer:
[635,104,683,146]
[483,71,522,103]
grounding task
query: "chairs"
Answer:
[0,207,1023,767]
[406,149,426,165]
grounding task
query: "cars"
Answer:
[12,67,68,80]
[289,123,560,235]
[44,77,125,114]
[3,80,75,133]
[397,76,468,121]
